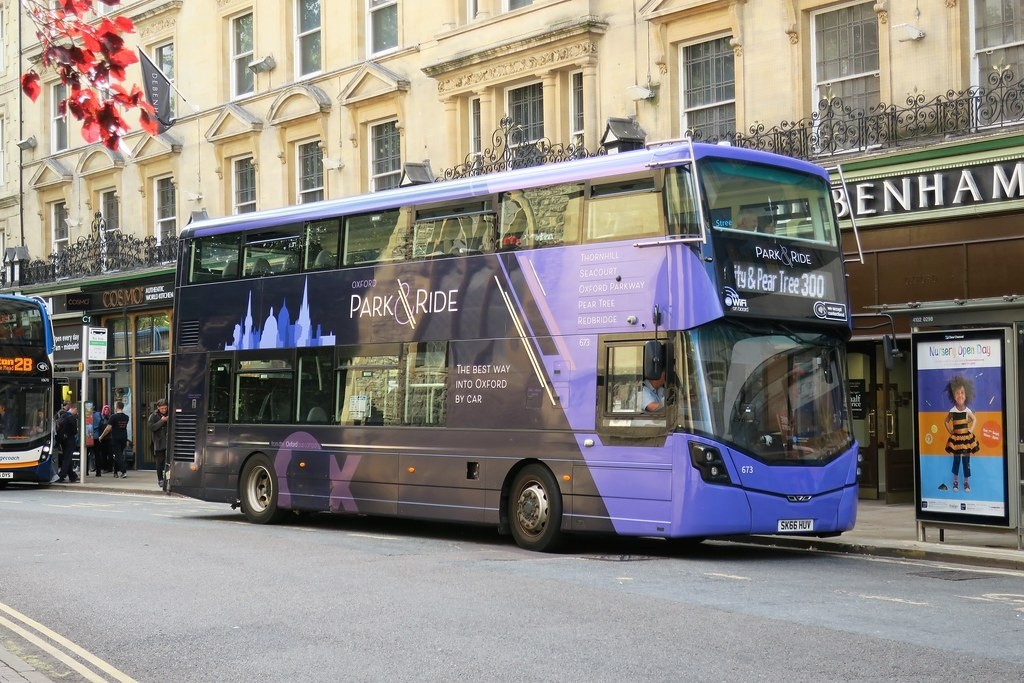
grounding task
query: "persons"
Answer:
[944,375,980,492]
[638,371,666,411]
[147,399,168,487]
[0,404,16,432]
[32,401,129,482]
[732,209,776,234]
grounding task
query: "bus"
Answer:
[162,137,900,550]
[0,294,72,488]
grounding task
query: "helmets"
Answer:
[644,341,666,380]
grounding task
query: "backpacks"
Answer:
[55,414,72,444]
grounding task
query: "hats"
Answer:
[157,399,168,405]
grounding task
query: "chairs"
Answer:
[218,231,505,278]
[214,371,444,429]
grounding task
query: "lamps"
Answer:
[247,55,276,76]
[321,157,344,170]
[627,83,654,102]
[65,218,81,227]
[184,190,203,201]
[16,135,37,151]
[892,23,925,43]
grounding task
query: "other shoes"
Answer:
[120,473,127,478]
[953,481,959,492]
[113,474,119,478]
[964,481,970,493]
[55,477,65,482]
[158,480,164,487]
[69,476,78,482]
